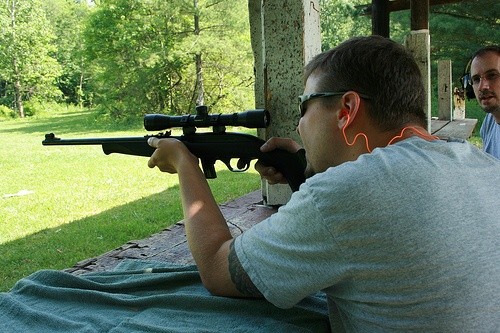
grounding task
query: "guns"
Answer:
[42,105,308,194]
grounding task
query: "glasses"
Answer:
[296,91,372,117]
[469,71,500,84]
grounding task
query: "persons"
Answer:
[145,33,500,333]
[466,45,500,159]
